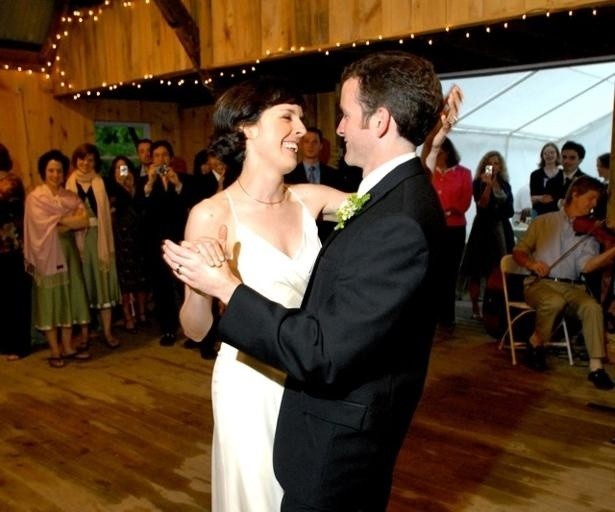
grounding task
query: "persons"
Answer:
[2,137,243,366]
[284,127,347,247]
[430,135,614,390]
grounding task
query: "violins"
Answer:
[574,216,615,250]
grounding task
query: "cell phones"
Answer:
[120,165,128,176]
[485,165,493,177]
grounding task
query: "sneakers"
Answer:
[442,334,463,347]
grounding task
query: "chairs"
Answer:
[498,254,575,366]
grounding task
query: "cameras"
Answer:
[157,165,167,174]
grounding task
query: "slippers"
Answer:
[105,337,121,348]
[48,337,91,367]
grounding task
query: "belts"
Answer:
[544,276,584,285]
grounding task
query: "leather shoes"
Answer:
[589,367,613,390]
[125,318,137,334]
[472,311,483,320]
[527,338,548,371]
[160,333,176,346]
[139,313,147,323]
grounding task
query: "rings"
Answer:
[176,264,182,273]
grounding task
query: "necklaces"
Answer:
[237,178,287,205]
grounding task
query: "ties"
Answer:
[216,177,224,193]
[563,177,570,190]
[308,166,315,183]
